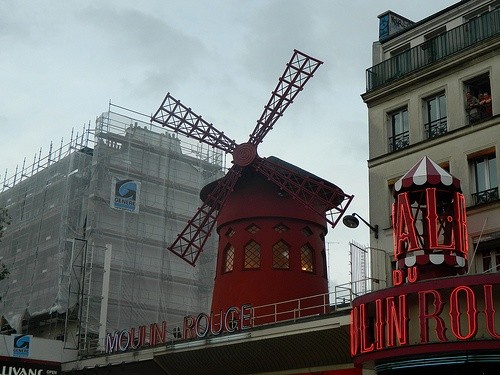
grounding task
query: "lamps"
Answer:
[344,214,360,229]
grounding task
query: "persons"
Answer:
[479,91,492,117]
[465,91,479,123]
